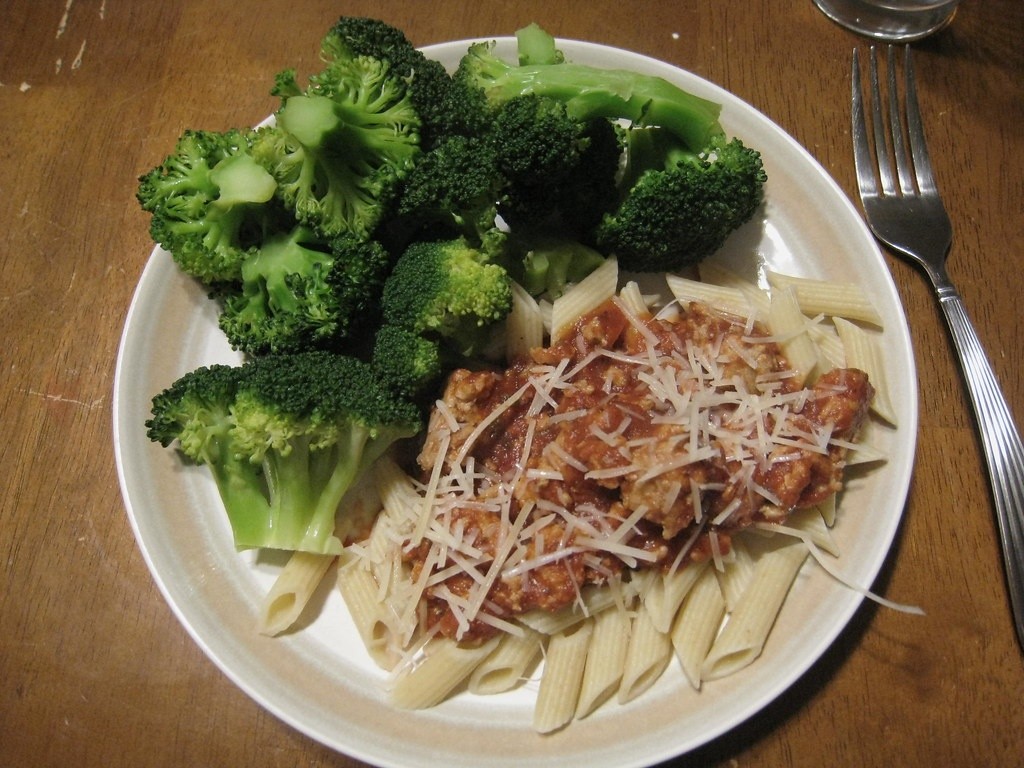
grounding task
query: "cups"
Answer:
[813,0,959,43]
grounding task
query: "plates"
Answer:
[113,36,918,768]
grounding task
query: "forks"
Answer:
[852,44,1024,660]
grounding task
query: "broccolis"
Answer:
[136,16,768,553]
